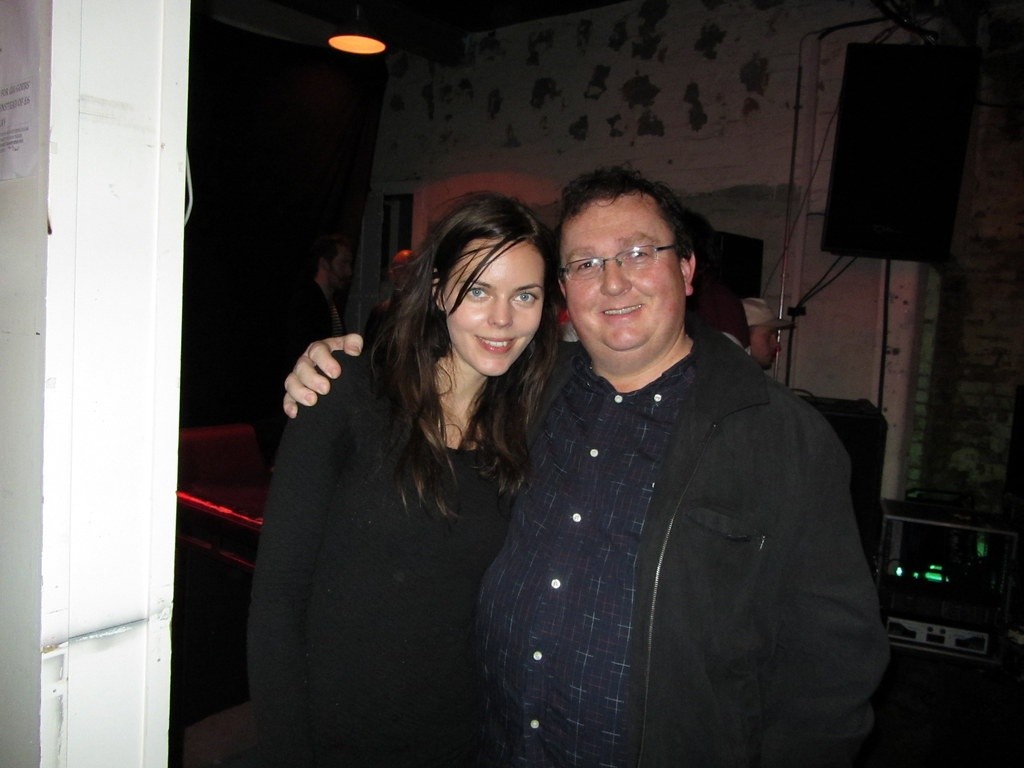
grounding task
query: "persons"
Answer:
[682,212,794,369]
[284,233,352,376]
[284,164,890,768]
[245,190,558,768]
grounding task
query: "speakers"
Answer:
[821,43,982,264]
[797,395,887,570]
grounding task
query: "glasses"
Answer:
[557,245,678,279]
